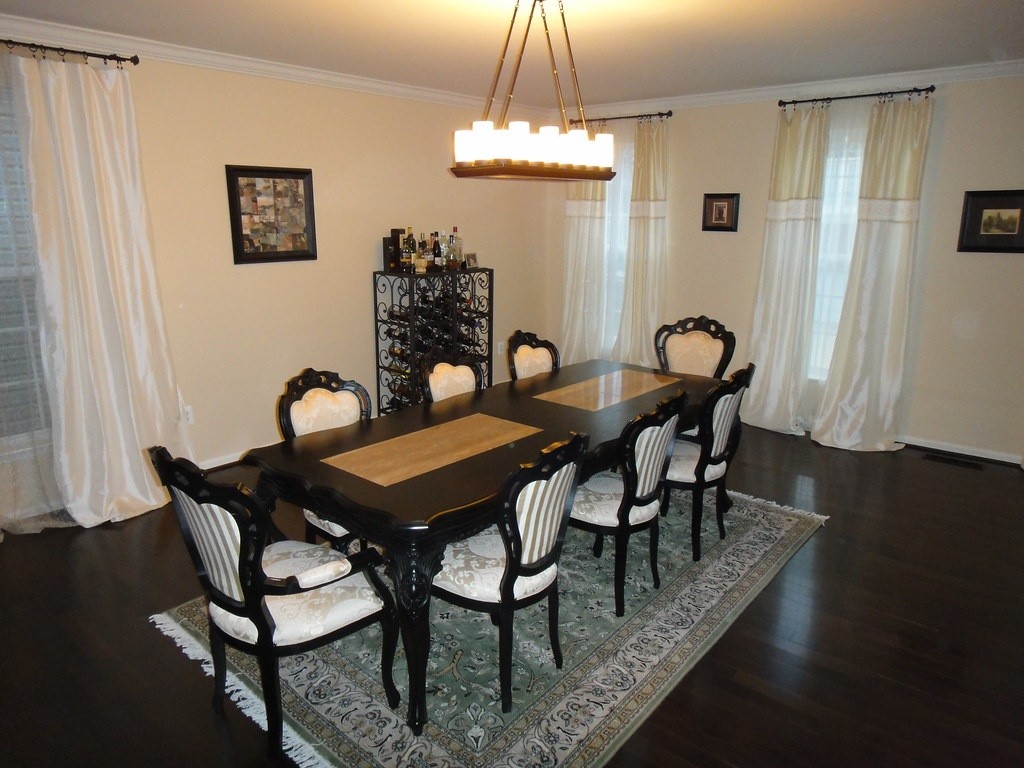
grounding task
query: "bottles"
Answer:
[447,227,464,266]
[439,230,450,273]
[399,226,416,274]
[419,231,442,269]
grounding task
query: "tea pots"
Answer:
[446,259,462,272]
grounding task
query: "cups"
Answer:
[414,258,428,274]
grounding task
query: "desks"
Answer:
[239,359,731,736]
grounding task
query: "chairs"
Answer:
[655,315,736,443]
[432,431,590,714]
[507,330,560,380]
[278,368,388,559]
[147,445,401,767]
[660,362,757,562]
[420,345,484,403]
[568,388,691,617]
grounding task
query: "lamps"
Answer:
[450,0,617,180]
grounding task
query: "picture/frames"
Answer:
[465,253,478,269]
[226,164,317,264]
[958,189,1024,253]
[702,193,740,231]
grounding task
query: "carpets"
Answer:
[148,461,831,768]
